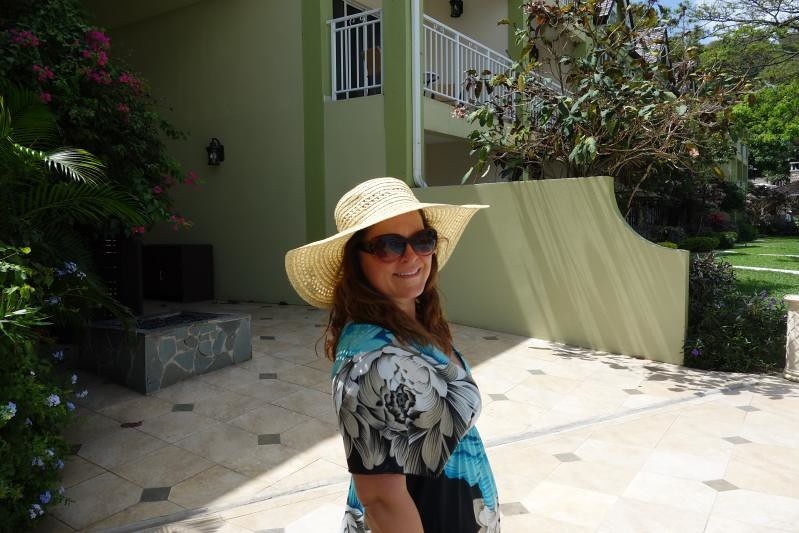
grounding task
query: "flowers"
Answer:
[0,244,89,533]
[0,0,211,240]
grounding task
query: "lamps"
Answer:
[205,138,224,166]
[449,0,463,17]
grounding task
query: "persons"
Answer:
[283,175,501,533]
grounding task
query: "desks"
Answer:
[422,71,440,99]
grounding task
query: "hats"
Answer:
[285,176,490,310]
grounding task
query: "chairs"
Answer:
[360,45,382,77]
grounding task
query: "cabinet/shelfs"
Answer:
[140,242,214,303]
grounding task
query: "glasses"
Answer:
[358,229,437,263]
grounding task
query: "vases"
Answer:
[95,225,121,320]
